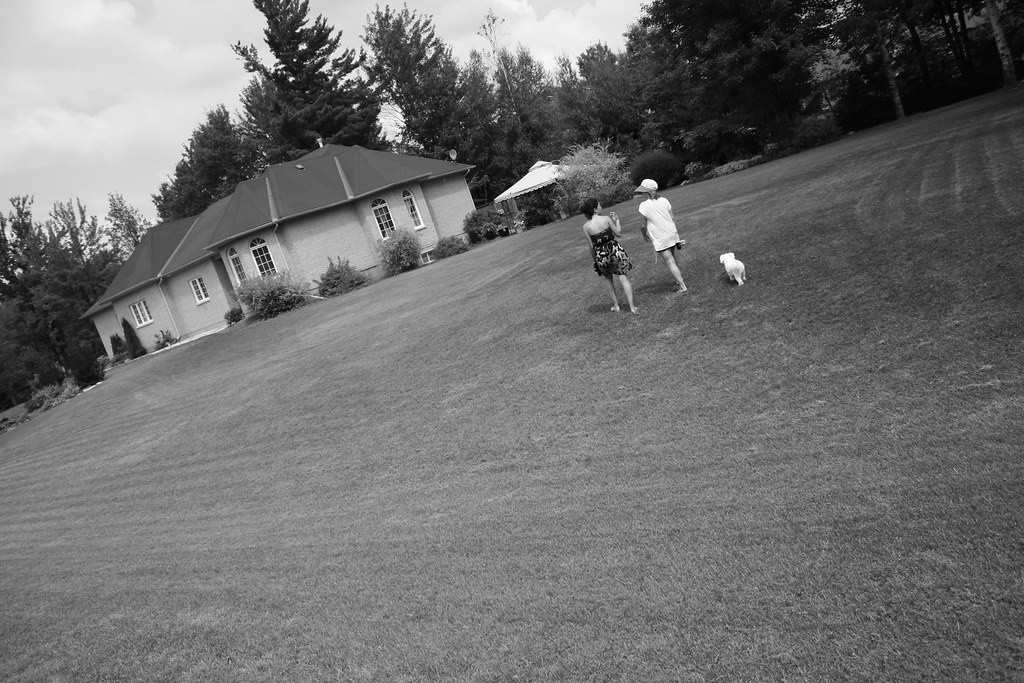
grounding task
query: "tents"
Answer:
[493,160,596,234]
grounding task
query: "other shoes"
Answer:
[675,288,688,294]
[610,306,620,313]
[631,307,638,313]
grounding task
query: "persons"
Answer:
[633,178,686,293]
[582,199,638,313]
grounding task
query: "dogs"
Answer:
[719,252,746,286]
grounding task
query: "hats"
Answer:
[634,179,658,192]
